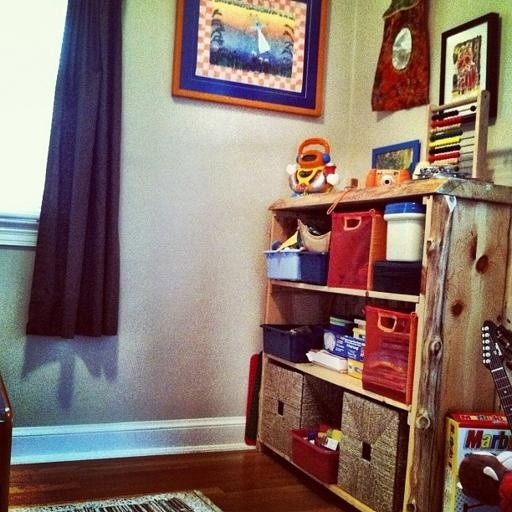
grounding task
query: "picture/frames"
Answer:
[172,1,328,118]
[372,141,419,178]
[439,11,500,122]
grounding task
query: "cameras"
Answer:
[365,169,411,188]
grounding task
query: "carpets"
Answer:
[8,488,225,512]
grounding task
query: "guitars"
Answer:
[480,320,512,433]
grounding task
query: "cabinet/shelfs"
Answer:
[257,178,512,512]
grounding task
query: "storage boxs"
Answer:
[258,362,408,512]
[264,251,327,285]
[262,323,323,364]
